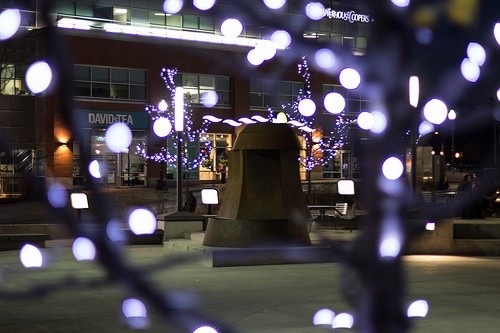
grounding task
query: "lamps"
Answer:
[337,178,356,195]
[201,187,219,205]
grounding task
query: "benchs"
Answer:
[306,203,358,232]
[421,189,455,207]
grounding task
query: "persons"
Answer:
[181,190,198,215]
[455,173,473,194]
[435,175,451,193]
